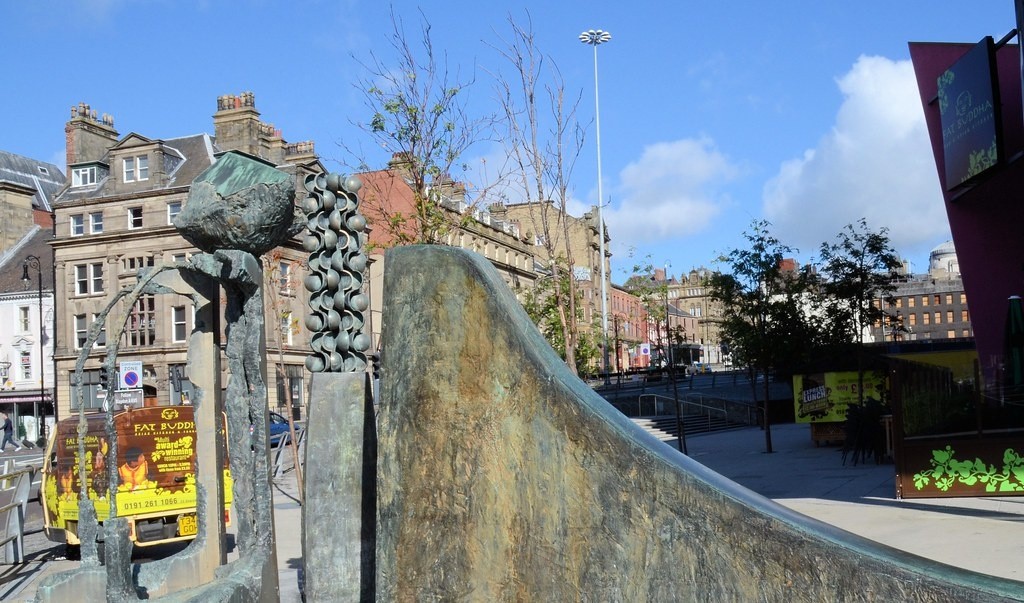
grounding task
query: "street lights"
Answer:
[578,28,614,387]
[20,254,47,449]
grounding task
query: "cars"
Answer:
[670,364,693,380]
[644,369,670,384]
[248,410,301,451]
[695,362,711,374]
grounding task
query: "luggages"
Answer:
[12,434,35,450]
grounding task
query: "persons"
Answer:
[59,460,75,500]
[117,447,152,489]
[92,438,107,498]
[0,412,36,452]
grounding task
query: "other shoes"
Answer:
[15,446,21,451]
[0,449,4,452]
